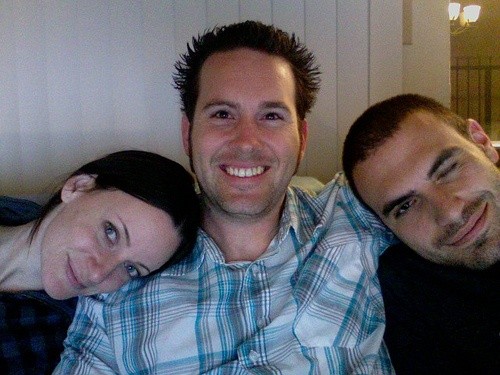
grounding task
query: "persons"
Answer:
[0,151,202,375]
[49,20,403,375]
[341,92,500,373]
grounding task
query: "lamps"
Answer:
[449,1,481,38]
